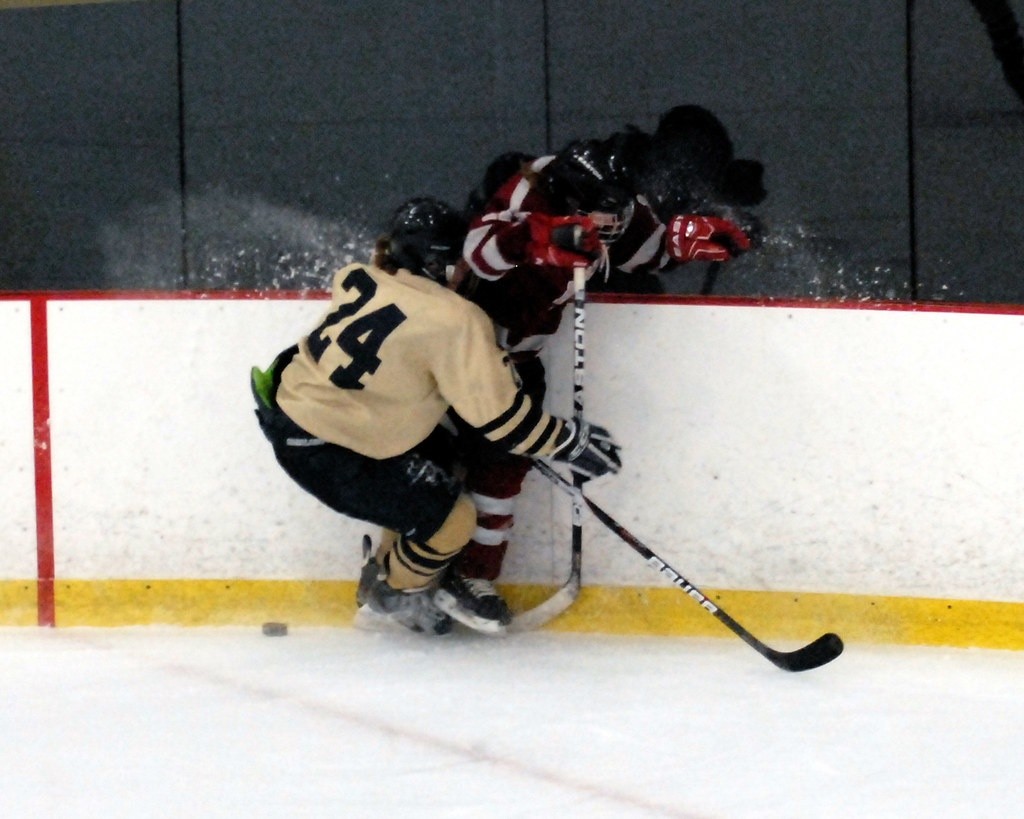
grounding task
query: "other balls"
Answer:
[262,621,287,637]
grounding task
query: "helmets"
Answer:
[539,138,634,247]
[389,196,461,285]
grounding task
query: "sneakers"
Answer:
[433,553,512,634]
[355,534,383,606]
[355,551,453,636]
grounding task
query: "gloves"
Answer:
[665,214,750,262]
[549,416,621,482]
[530,211,602,267]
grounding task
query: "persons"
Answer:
[434,138,751,634]
[250,196,623,636]
[586,103,768,296]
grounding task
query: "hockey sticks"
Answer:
[508,221,588,632]
[527,456,843,673]
